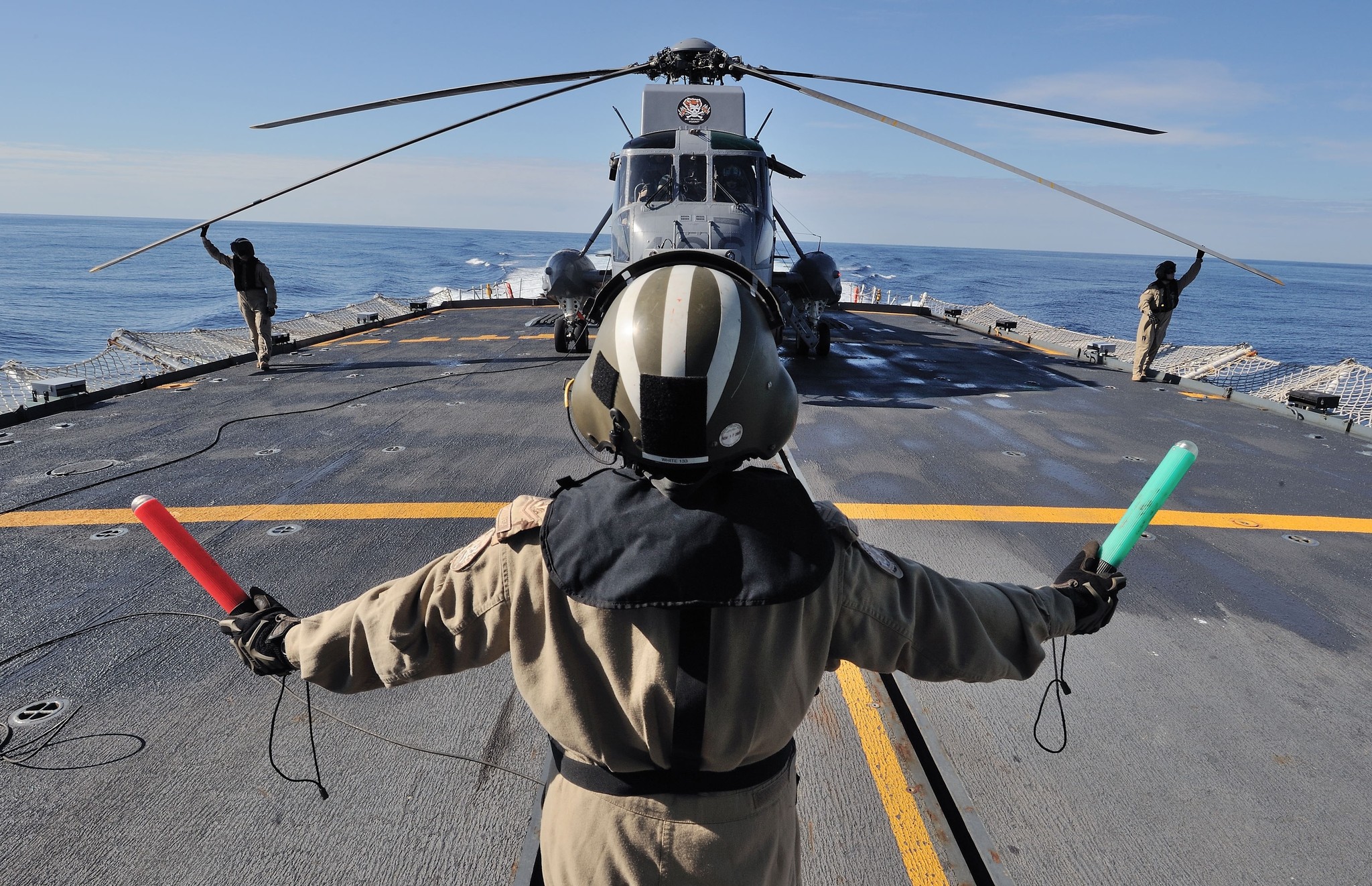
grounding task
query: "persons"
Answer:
[222,249,1125,886]
[200,224,278,370]
[1131,245,1205,381]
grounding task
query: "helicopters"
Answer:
[88,37,1285,353]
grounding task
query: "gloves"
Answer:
[1049,541,1127,635]
[200,224,210,237]
[219,586,302,677]
[1148,311,1160,324]
[1196,245,1205,259]
[269,307,275,317]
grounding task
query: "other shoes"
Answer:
[257,362,260,367]
[259,361,269,370]
[1142,370,1146,375]
[1131,375,1141,381]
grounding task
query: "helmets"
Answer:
[570,264,799,470]
[230,237,255,258]
[1155,261,1175,280]
